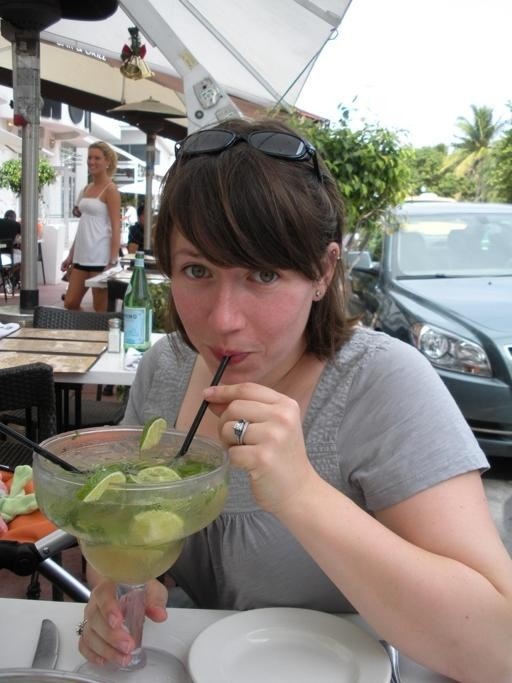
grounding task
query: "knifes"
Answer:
[29,618,61,669]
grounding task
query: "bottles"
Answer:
[123,251,152,352]
[108,319,121,353]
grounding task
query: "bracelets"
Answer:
[108,258,118,266]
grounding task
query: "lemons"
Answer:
[132,465,178,487]
[139,417,167,453]
[127,509,182,546]
[77,469,125,504]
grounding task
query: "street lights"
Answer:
[106,97,187,253]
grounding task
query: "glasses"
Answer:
[174,130,322,181]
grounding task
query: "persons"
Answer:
[76,122,512,683]
[127,204,147,254]
[61,141,120,313]
[0,210,20,294]
[120,202,138,226]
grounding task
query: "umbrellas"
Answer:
[117,178,164,195]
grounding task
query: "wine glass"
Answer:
[33,426,226,683]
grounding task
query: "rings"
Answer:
[76,616,87,637]
[232,417,249,446]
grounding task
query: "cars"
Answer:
[340,192,512,459]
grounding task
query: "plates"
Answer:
[187,606,392,683]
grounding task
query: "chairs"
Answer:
[36,240,48,285]
[0,238,16,300]
[106,278,128,311]
[0,361,79,603]
[1,304,127,438]
